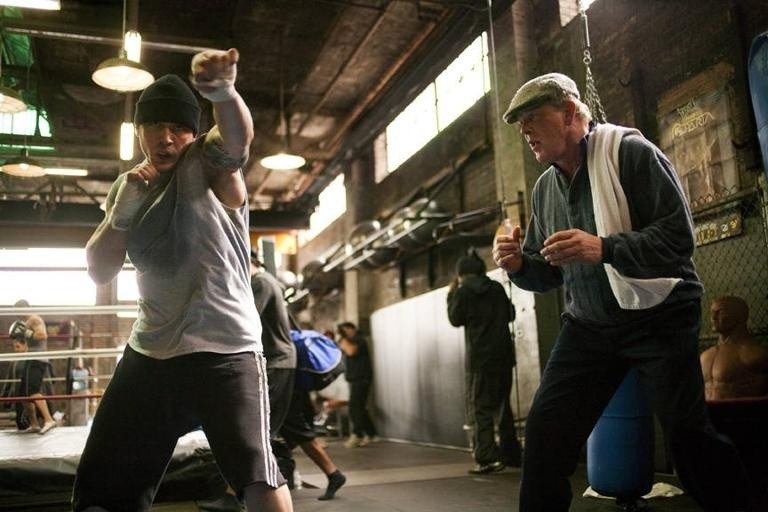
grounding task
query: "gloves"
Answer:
[8,322,33,338]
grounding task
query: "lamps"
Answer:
[116,89,135,161]
[258,80,307,171]
[122,0,142,62]
[88,0,153,96]
[0,125,44,178]
[0,82,29,117]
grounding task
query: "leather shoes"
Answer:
[469,460,503,474]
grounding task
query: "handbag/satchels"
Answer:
[290,329,347,392]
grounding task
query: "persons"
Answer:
[491,69,768,510]
[194,253,347,512]
[446,251,524,475]
[8,298,56,433]
[0,336,29,430]
[701,295,768,403]
[334,320,375,449]
[71,47,298,510]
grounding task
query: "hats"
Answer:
[504,73,579,124]
[455,252,489,276]
[135,73,201,136]
